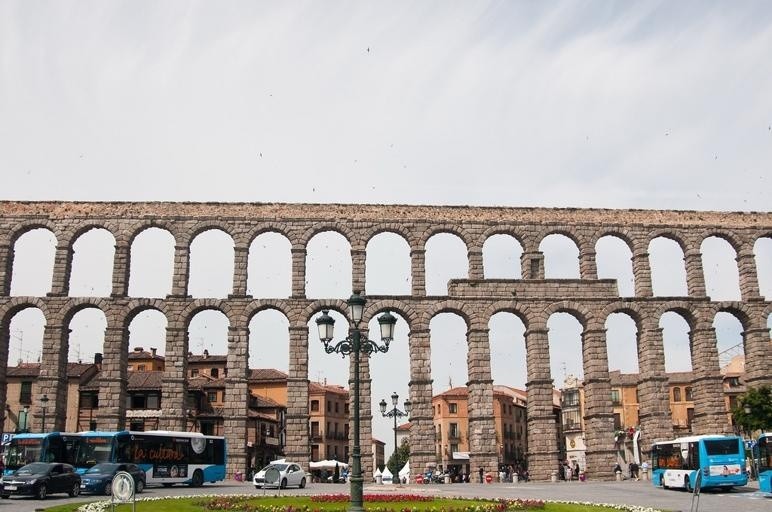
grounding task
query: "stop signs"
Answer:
[416,475,423,484]
[486,475,492,482]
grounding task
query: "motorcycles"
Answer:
[424,469,444,484]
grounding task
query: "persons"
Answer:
[719,465,729,475]
[443,460,651,482]
[744,456,753,481]
[315,467,322,482]
[322,467,329,483]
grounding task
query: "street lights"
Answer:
[315,289,398,507]
[379,391,414,484]
[40,394,50,433]
[23,405,30,431]
[743,402,753,439]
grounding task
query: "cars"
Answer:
[253,462,306,489]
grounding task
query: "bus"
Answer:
[0,428,228,500]
[750,432,772,496]
[650,433,748,493]
